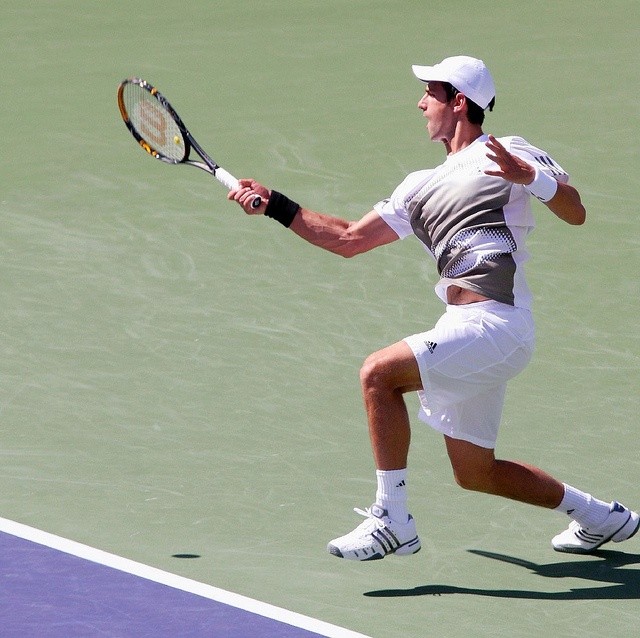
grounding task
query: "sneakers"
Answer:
[327,503,421,560]
[551,501,639,554]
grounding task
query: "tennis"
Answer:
[173,136,181,145]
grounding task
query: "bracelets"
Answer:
[523,165,557,204]
[264,189,299,229]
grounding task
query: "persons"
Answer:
[226,56,640,561]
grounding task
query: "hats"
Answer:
[412,55,497,110]
